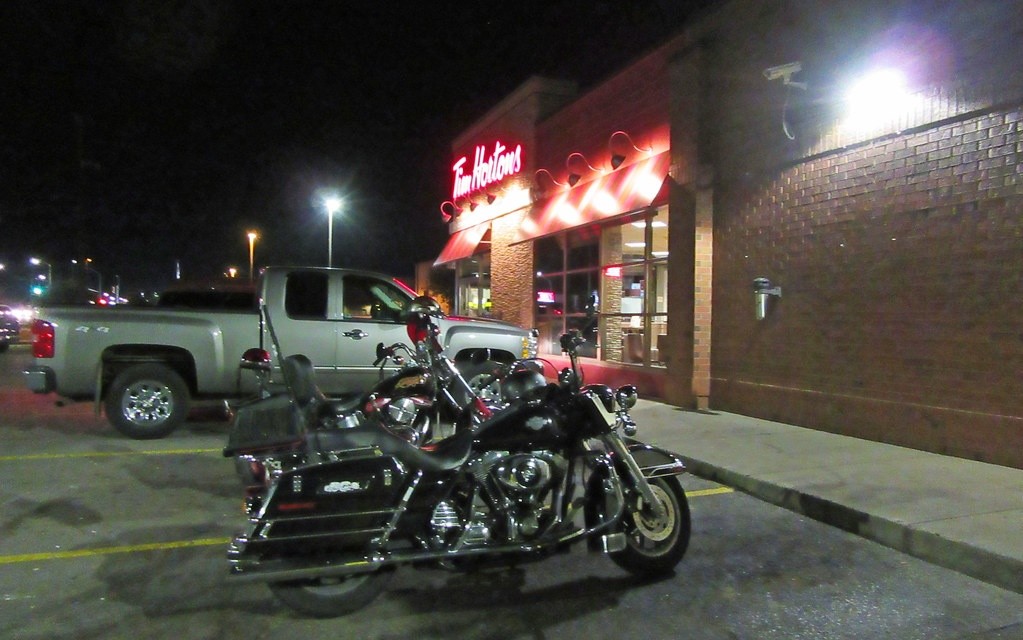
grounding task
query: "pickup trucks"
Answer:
[22,267,540,440]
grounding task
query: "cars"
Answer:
[0,304,21,353]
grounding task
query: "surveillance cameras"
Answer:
[760,62,807,92]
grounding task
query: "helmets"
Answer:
[499,369,548,403]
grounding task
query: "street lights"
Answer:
[249,233,256,284]
[326,200,336,267]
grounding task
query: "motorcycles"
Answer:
[224,314,693,618]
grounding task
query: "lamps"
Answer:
[752,277,782,321]
[565,153,606,188]
[484,177,505,205]
[467,188,486,213]
[608,131,654,170]
[440,201,456,224]
[453,193,470,218]
[530,168,566,202]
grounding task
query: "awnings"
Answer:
[508,149,669,247]
[432,220,492,266]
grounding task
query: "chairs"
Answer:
[628,333,644,363]
[657,334,666,366]
